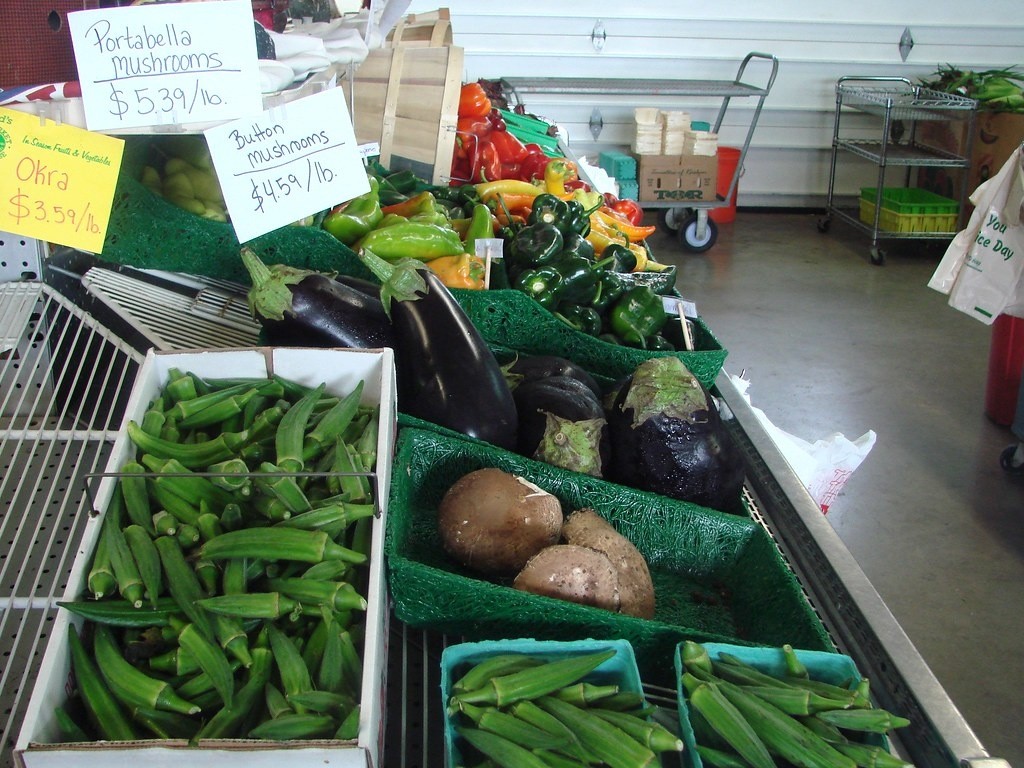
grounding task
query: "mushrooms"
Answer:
[438,468,657,620]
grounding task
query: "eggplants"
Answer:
[239,245,731,512]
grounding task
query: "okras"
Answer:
[54,364,378,742]
[444,640,918,768]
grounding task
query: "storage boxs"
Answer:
[676,642,893,768]
[447,108,729,394]
[93,142,385,295]
[860,187,958,213]
[858,197,958,237]
[439,635,653,768]
[384,426,837,671]
[12,347,399,768]
[986,312,1024,428]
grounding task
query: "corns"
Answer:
[921,64,1024,107]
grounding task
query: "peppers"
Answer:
[295,82,701,354]
[143,144,229,223]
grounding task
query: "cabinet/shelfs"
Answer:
[820,73,976,266]
[916,106,1024,226]
